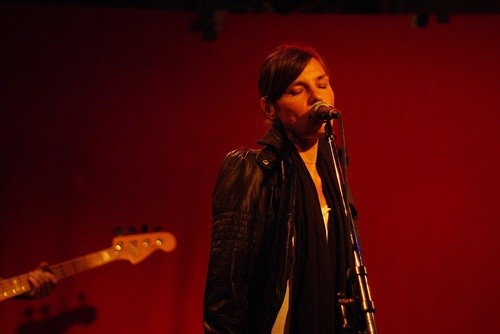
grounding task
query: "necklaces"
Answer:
[304,161,317,164]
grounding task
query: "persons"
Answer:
[0,261,59,300]
[203,45,369,334]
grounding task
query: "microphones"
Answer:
[311,101,342,121]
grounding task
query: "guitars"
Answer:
[0,223,177,301]
